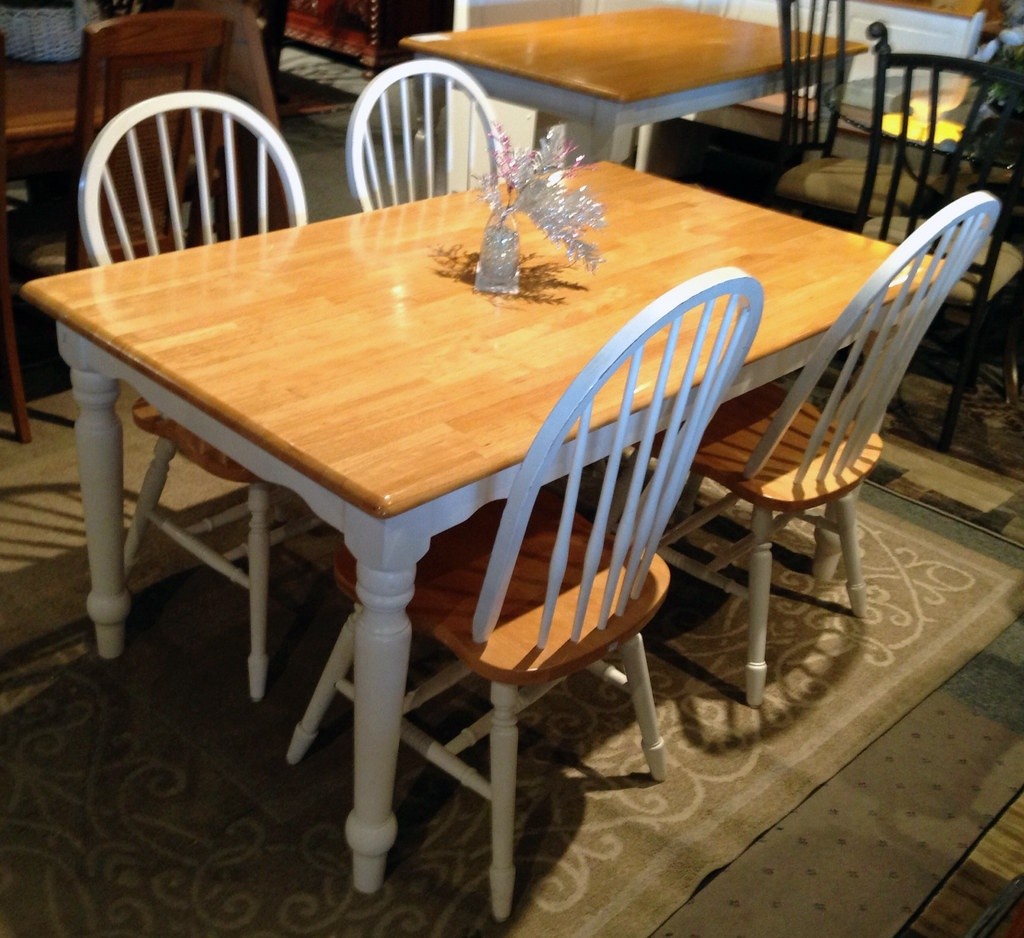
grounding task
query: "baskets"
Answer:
[0,0,99,62]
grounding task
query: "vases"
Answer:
[473,225,520,293]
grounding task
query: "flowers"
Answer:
[469,122,606,271]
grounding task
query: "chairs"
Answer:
[283,266,764,922]
[78,90,321,703]
[646,0,846,191]
[347,59,503,217]
[623,190,1002,710]
[854,22,1024,452]
[78,7,242,269]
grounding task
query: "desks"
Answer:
[20,160,948,894]
[398,5,869,161]
[0,28,105,444]
